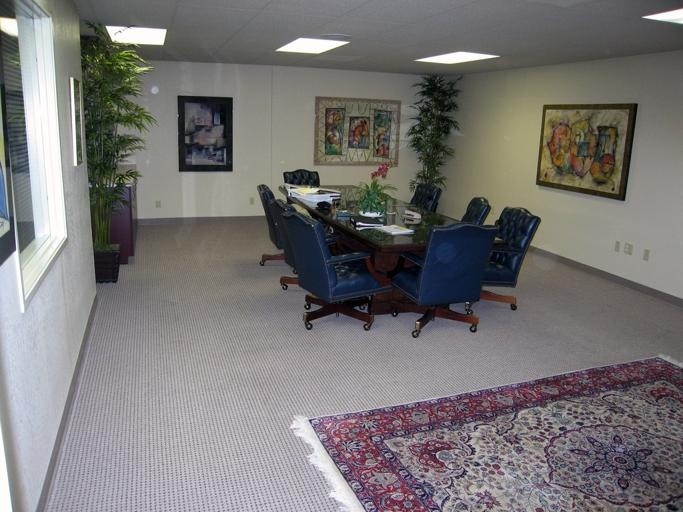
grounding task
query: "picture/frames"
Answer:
[0,84,16,268]
[314,97,401,168]
[534,102,638,201]
[176,94,233,173]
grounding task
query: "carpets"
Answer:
[288,354,683,512]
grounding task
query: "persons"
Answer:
[375,128,389,157]
[326,111,340,146]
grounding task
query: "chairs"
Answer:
[405,184,443,213]
[280,168,322,189]
[268,198,339,289]
[277,208,394,333]
[477,206,542,314]
[457,196,490,224]
[389,220,499,337]
[256,183,285,265]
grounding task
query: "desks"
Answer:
[276,183,467,315]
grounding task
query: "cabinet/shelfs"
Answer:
[87,163,139,266]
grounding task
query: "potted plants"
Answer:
[338,180,398,218]
[79,23,157,281]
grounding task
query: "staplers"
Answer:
[404,210,422,219]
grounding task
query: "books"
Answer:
[349,216,382,228]
[286,184,341,209]
[377,223,415,236]
